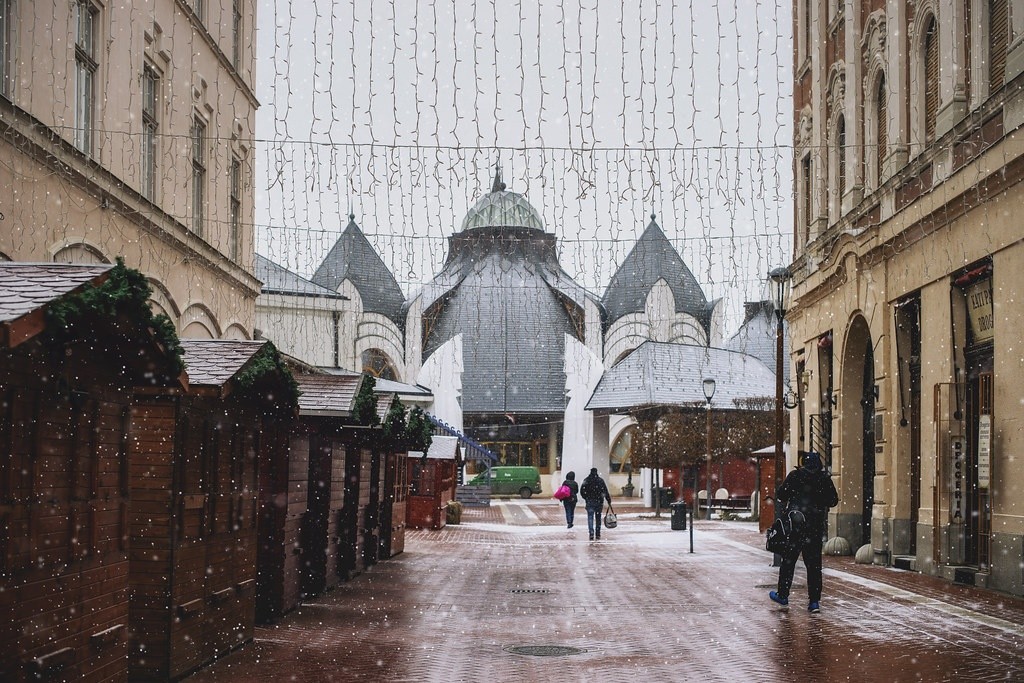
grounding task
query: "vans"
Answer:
[466,466,543,500]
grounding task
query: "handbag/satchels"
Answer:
[604,505,617,528]
[766,520,807,557]
[553,480,570,499]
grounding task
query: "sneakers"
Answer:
[769,591,788,605]
[808,602,820,613]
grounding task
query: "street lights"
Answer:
[767,267,794,567]
[701,377,716,520]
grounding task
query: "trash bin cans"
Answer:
[670,502,687,529]
[651,487,673,508]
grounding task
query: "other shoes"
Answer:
[568,524,574,528]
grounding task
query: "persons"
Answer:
[560,471,578,528]
[580,468,611,540]
[770,453,839,613]
